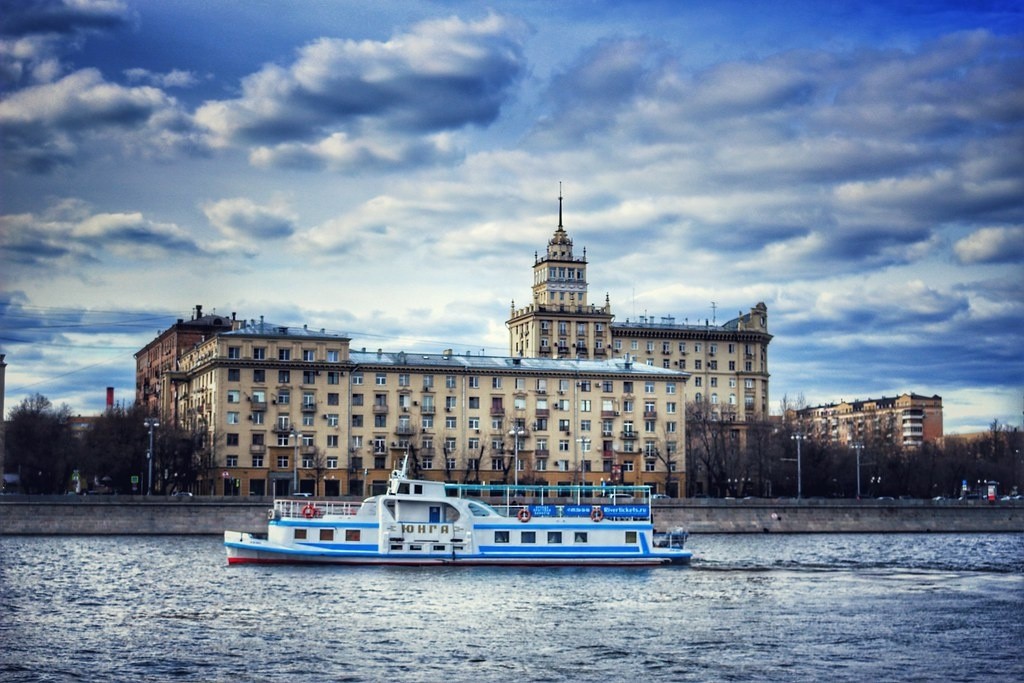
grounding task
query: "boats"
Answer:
[225,452,691,568]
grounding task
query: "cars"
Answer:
[292,492,313,498]
[175,492,193,496]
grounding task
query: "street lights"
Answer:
[144,417,160,495]
[289,429,303,492]
[787,432,809,498]
[849,442,866,498]
[507,426,525,485]
[577,438,591,485]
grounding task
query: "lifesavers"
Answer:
[517,509,530,522]
[591,508,603,522]
[302,505,315,519]
